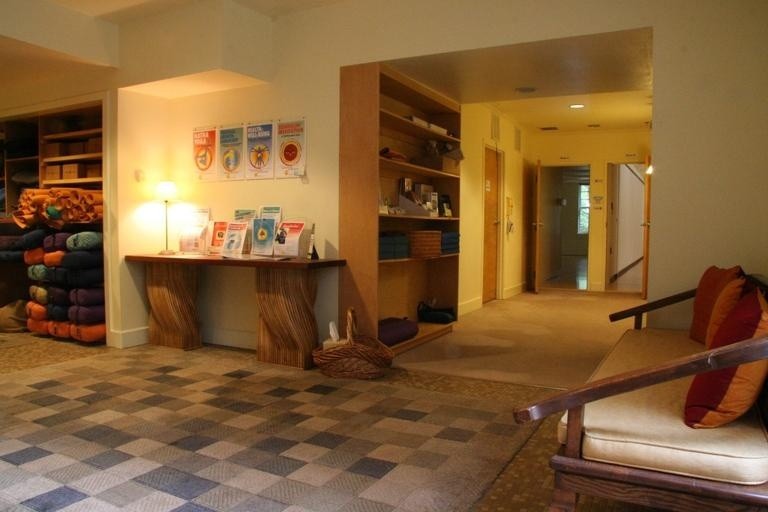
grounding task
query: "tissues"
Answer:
[322,320,347,351]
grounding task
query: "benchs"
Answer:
[512,277,768,511]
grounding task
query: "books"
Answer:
[398,175,439,211]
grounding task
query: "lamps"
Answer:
[156,181,179,255]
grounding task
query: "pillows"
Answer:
[683,263,768,430]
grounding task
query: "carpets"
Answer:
[1,349,545,512]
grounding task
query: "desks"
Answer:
[125,255,346,371]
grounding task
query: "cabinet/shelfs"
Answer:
[1,105,105,217]
[339,64,462,350]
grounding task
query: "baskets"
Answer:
[312,307,394,379]
[379,230,459,259]
